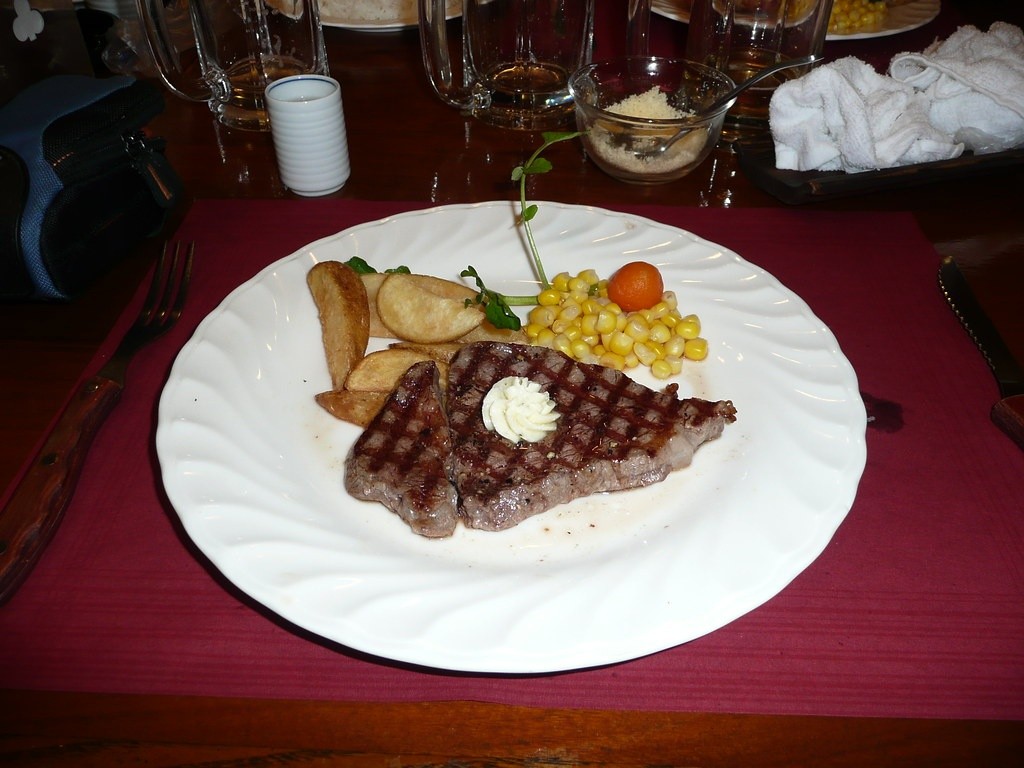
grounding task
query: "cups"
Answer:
[418,0,594,131]
[135,0,329,132]
[686,0,834,143]
[265,74,351,197]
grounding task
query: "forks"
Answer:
[0,238,195,607]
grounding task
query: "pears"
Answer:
[307,262,520,427]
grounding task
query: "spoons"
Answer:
[599,55,824,159]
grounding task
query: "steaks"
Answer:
[346,338,737,539]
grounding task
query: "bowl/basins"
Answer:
[568,57,739,185]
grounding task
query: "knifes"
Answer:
[937,256,1024,443]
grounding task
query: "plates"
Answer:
[266,0,491,32]
[649,0,941,40]
[156,201,868,675]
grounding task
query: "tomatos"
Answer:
[606,261,664,312]
